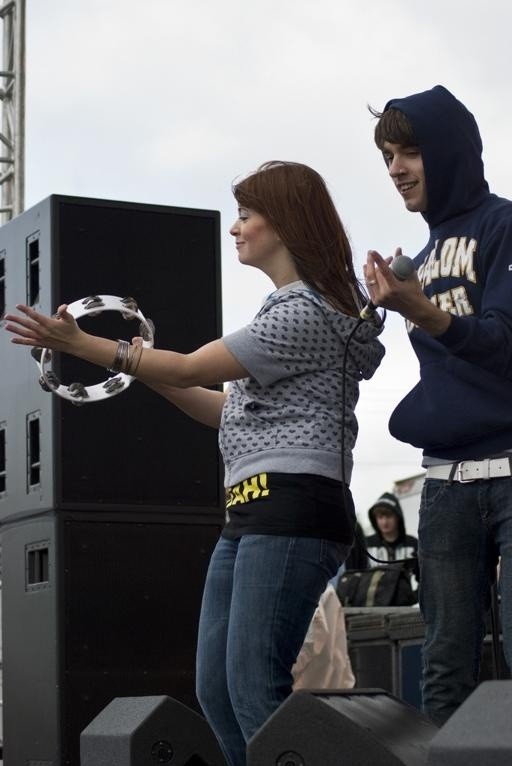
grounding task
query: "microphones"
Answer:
[357,254,416,328]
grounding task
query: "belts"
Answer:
[421,456,512,484]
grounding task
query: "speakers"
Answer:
[107,430,115,434]
[427,680,512,765]
[1,194,224,525]
[79,693,224,766]
[246,686,440,765]
[1,508,227,766]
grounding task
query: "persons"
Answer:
[362,86,512,730]
[363,493,420,606]
[4,160,383,766]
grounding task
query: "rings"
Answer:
[366,280,377,287]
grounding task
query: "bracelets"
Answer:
[109,337,143,377]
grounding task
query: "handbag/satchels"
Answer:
[336,565,417,612]
